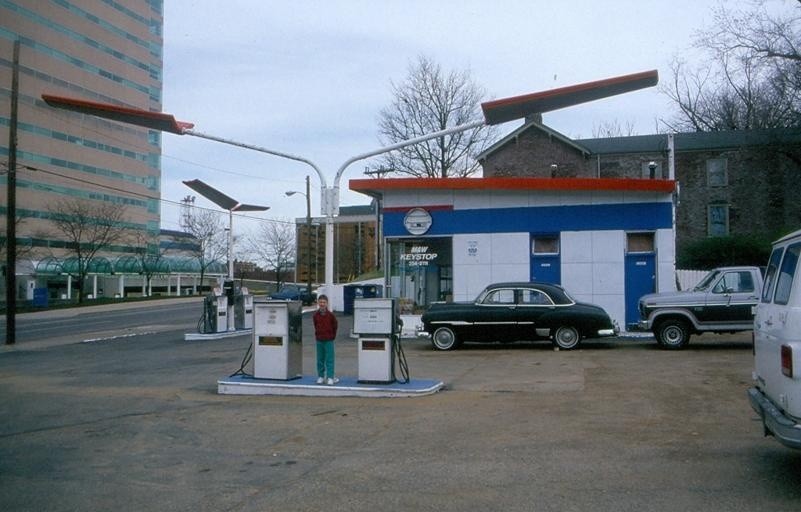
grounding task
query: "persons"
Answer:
[313,295,338,385]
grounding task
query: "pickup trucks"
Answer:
[637,263,768,348]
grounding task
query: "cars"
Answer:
[312,285,321,303]
[417,281,622,351]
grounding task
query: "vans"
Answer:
[744,225,801,451]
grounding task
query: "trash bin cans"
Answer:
[33,288,48,308]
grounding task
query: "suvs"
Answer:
[267,285,311,307]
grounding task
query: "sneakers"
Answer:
[317,377,324,384]
[327,378,334,385]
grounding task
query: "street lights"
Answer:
[224,227,230,275]
[286,174,313,306]
